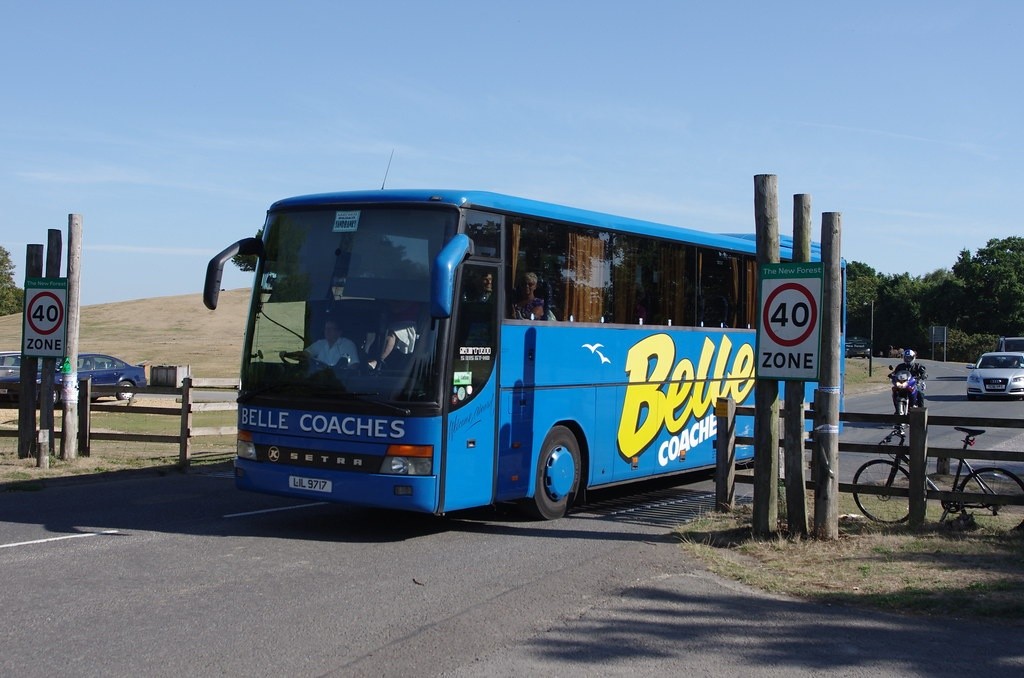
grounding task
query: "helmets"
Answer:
[903,349,916,365]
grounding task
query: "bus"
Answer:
[199,186,849,520]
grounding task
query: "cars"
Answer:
[965,351,1024,400]
[845,335,871,358]
[0,351,43,378]
[0,353,147,408]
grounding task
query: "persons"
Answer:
[685,260,735,327]
[634,292,647,324]
[1009,358,1019,367]
[887,349,929,408]
[512,271,545,320]
[364,293,419,371]
[279,318,361,370]
[987,358,995,367]
[466,272,494,304]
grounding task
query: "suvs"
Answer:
[996,336,1024,352]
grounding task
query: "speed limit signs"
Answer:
[21,276,70,359]
[752,261,825,382]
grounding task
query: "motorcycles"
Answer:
[886,364,928,429]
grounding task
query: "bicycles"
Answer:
[851,425,1024,532]
